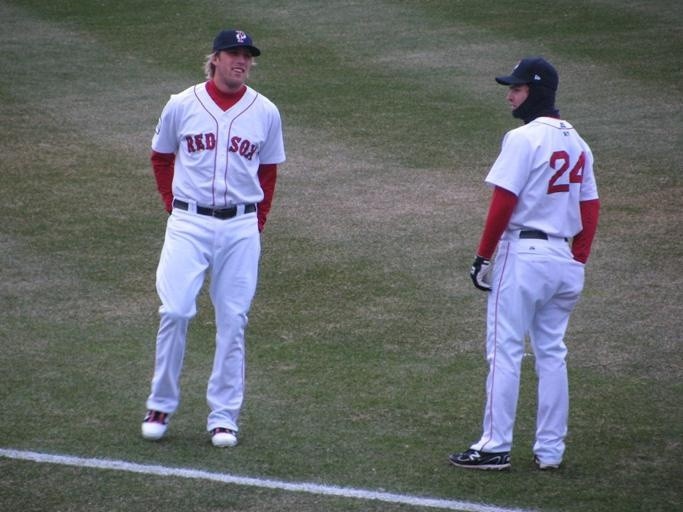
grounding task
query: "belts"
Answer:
[173,198,257,219]
[518,231,567,242]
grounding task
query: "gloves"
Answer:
[467,254,492,292]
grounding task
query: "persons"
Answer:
[139,29,286,449]
[447,56,600,472]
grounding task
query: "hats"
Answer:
[212,28,261,57]
[493,55,558,91]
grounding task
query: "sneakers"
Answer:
[533,452,560,470]
[209,426,239,448]
[140,409,170,441]
[448,449,511,470]
